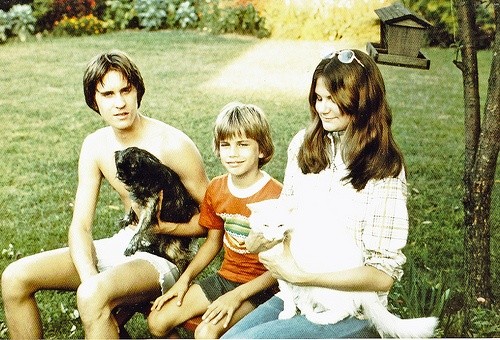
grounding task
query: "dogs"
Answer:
[115,147,202,281]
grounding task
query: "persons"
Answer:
[212,48,409,338]
[143,102,287,339]
[0,50,209,340]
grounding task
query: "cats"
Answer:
[244,197,440,339]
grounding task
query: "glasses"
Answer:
[321,49,364,71]
